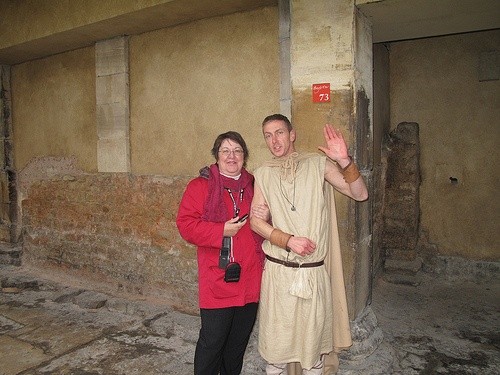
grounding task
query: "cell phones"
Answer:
[234,214,249,223]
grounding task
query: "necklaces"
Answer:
[277,162,305,213]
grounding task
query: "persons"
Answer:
[176,131,271,375]
[250,114,369,375]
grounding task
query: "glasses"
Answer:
[218,147,244,156]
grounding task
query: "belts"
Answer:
[266,255,324,268]
